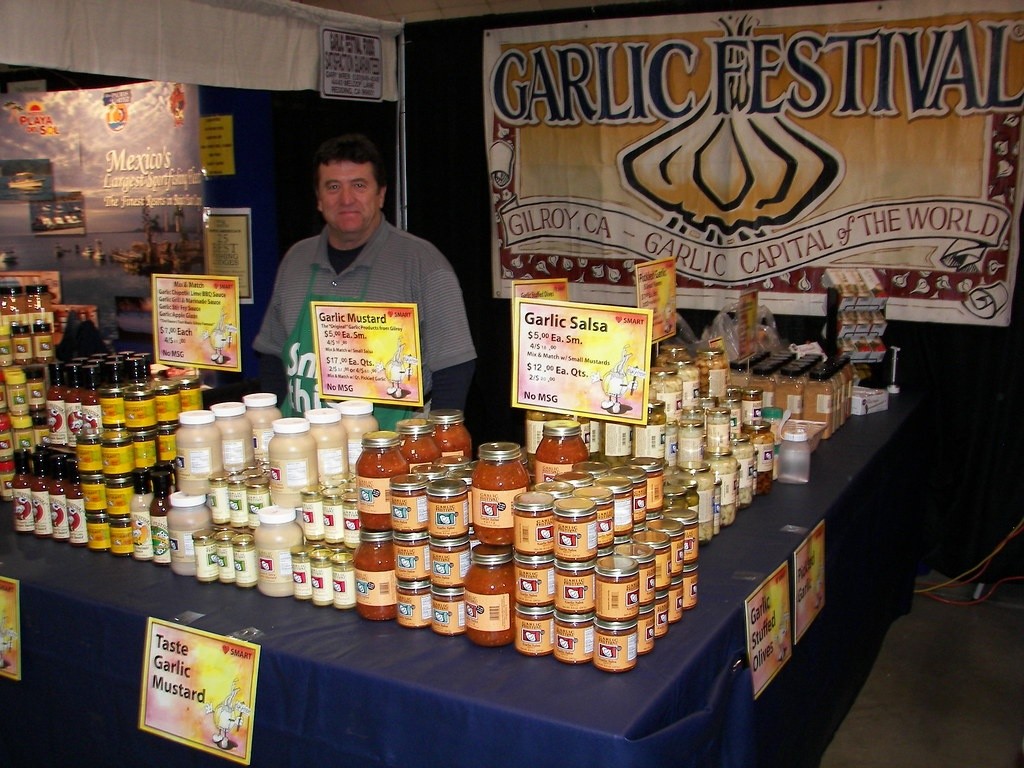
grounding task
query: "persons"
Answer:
[247,134,477,432]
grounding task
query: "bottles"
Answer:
[778,409,811,484]
[13,351,171,565]
